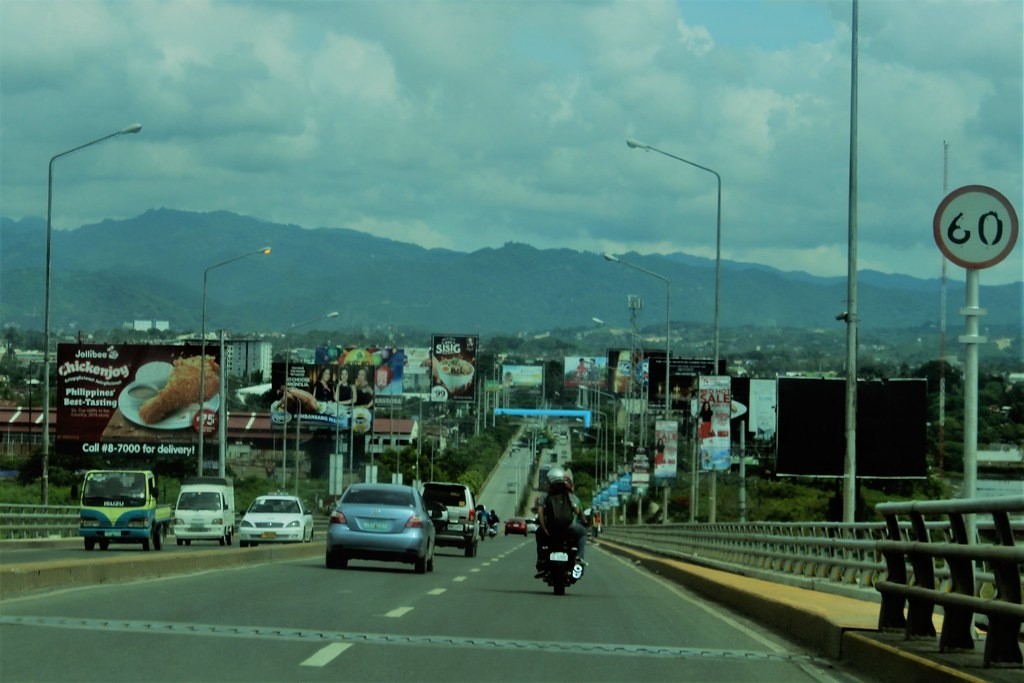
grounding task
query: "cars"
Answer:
[477,513,538,537]
[238,491,314,547]
[417,481,482,558]
[326,483,435,574]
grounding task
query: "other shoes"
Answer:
[534,569,547,578]
[542,576,549,582]
[575,559,589,568]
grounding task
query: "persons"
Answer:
[333,369,357,406]
[574,359,589,382]
[286,503,299,513]
[505,372,515,388]
[591,509,602,538]
[314,367,338,403]
[356,368,375,410]
[534,468,589,579]
[656,432,665,464]
[488,509,501,530]
[475,504,490,535]
[697,401,716,439]
[588,358,600,381]
[271,364,347,418]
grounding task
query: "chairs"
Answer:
[105,477,124,496]
[261,504,273,511]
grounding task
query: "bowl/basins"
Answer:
[126,383,159,410]
[437,358,476,389]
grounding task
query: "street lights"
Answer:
[583,358,627,525]
[42,123,142,536]
[197,246,272,477]
[591,317,644,524]
[579,385,616,526]
[626,139,721,526]
[282,312,339,489]
[576,405,608,525]
[603,252,671,523]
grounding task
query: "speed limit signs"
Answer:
[932,184,1019,272]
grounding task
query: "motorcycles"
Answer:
[478,516,499,541]
[531,508,592,595]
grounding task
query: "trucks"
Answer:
[78,469,172,551]
[173,477,235,546]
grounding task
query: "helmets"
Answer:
[484,505,487,510]
[565,468,575,491]
[547,467,568,484]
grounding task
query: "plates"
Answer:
[118,381,220,430]
